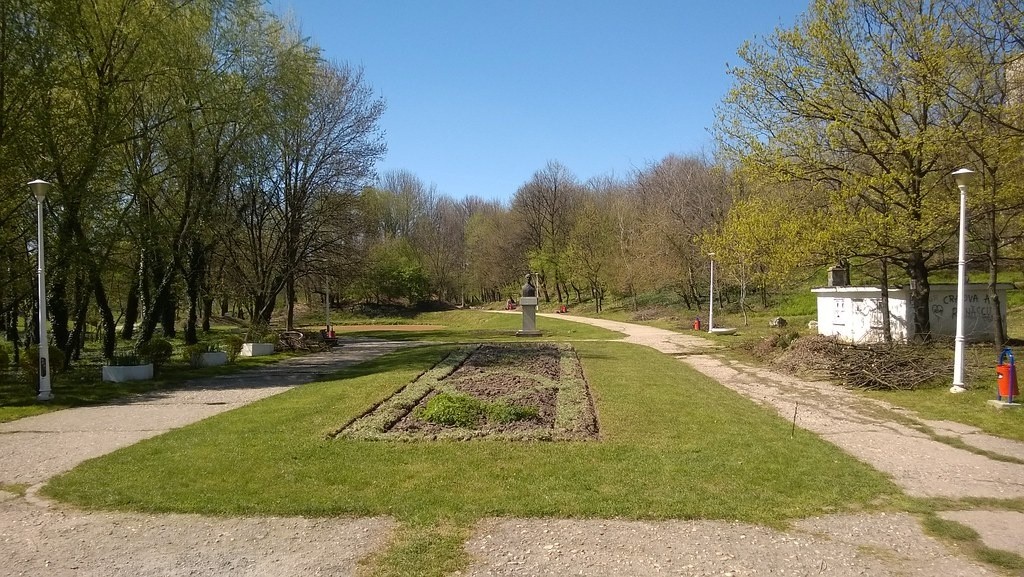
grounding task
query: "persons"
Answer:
[522,274,536,297]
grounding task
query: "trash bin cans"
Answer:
[329,325,335,338]
[694,317,701,331]
[561,304,567,313]
[996,348,1021,403]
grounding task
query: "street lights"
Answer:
[706,252,716,330]
[27,179,54,400]
[322,258,330,341]
[947,168,975,394]
[536,273,538,311]
